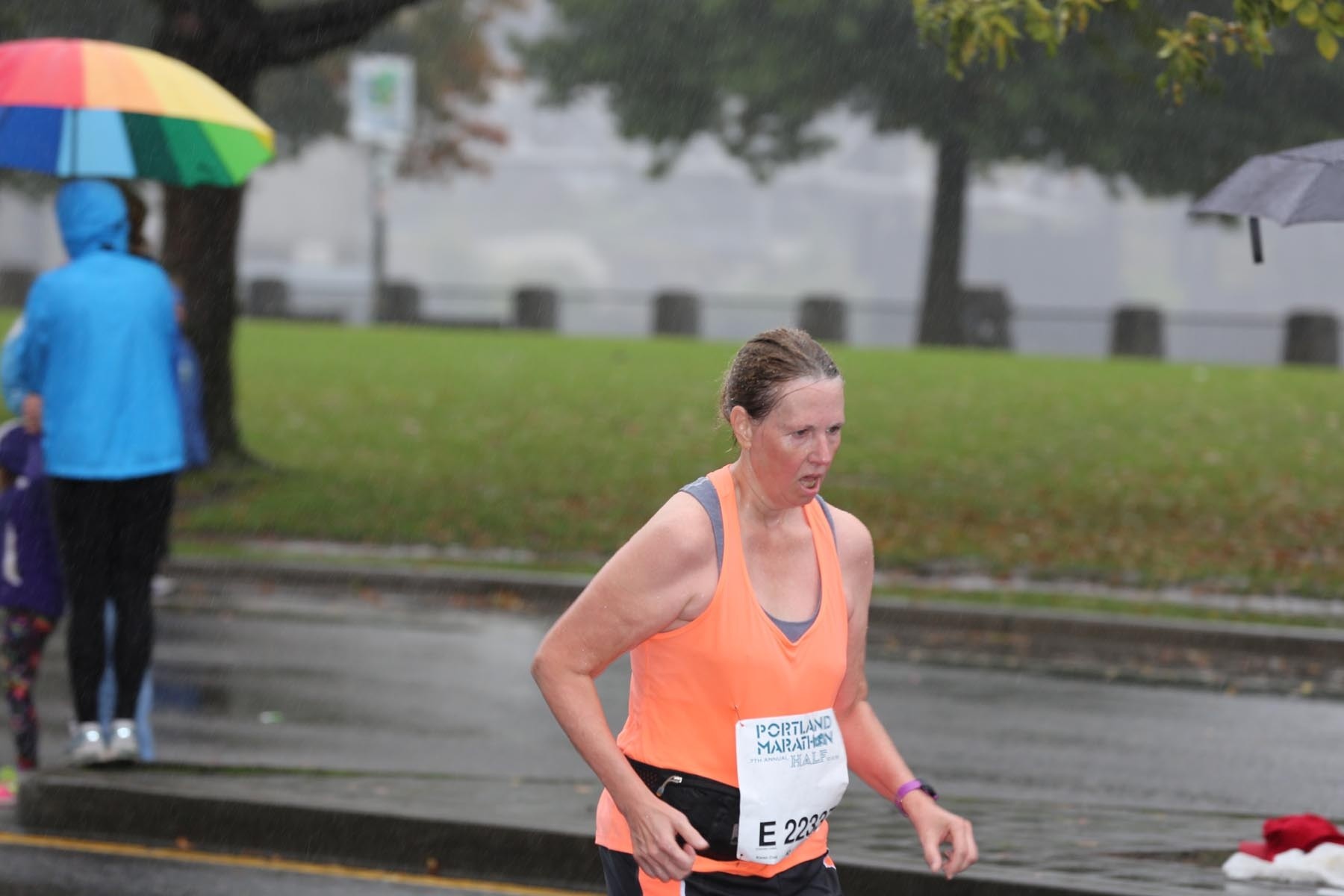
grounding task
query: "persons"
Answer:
[529,327,978,896]
[1,171,202,805]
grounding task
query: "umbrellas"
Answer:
[1188,138,1344,223]
[0,38,275,189]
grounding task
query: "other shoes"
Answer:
[107,717,143,761]
[63,717,107,769]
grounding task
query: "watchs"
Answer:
[892,776,940,817]
